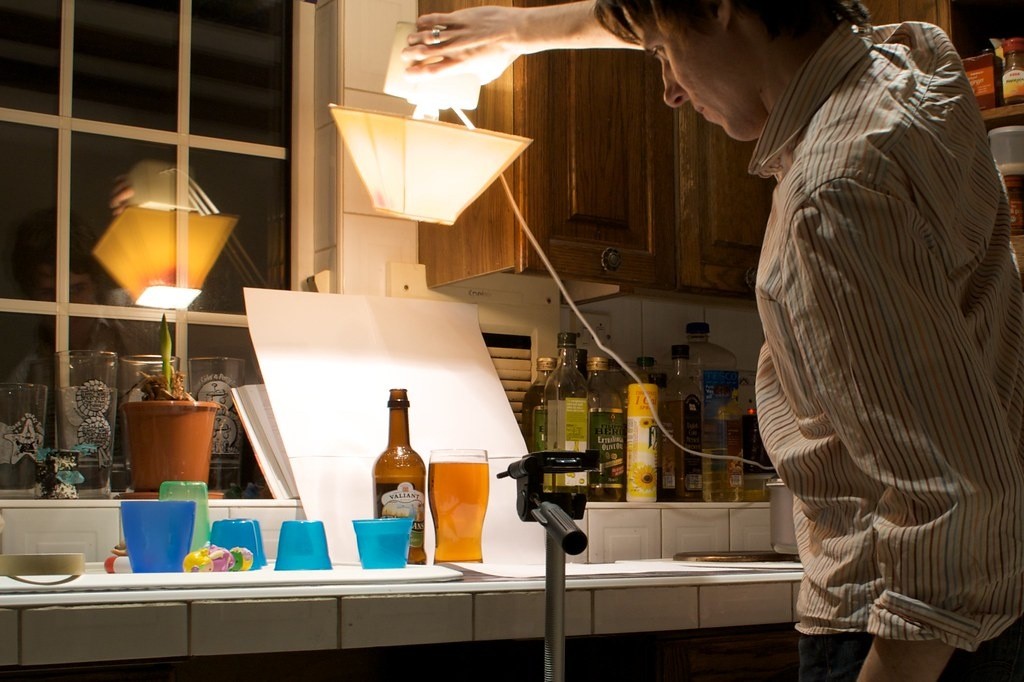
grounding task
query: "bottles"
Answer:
[373,388,426,564]
[766,479,798,555]
[578,322,772,501]
[105,555,132,575]
[521,357,555,452]
[543,332,590,502]
[1000,37,1024,104]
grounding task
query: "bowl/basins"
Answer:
[987,127,1024,175]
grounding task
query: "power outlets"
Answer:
[733,370,755,415]
[573,310,612,356]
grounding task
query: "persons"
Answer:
[401,0,1024,682]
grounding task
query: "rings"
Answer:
[432,29,440,44]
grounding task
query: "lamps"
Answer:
[91,160,239,308]
[326,20,534,227]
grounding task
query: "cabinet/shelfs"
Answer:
[418,0,1024,307]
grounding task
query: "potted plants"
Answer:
[117,312,220,492]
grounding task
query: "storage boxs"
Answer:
[960,53,1005,111]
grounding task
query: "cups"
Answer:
[0,350,247,498]
[157,481,209,553]
[211,519,267,570]
[274,521,332,571]
[351,519,414,569]
[120,501,195,573]
[428,450,490,564]
